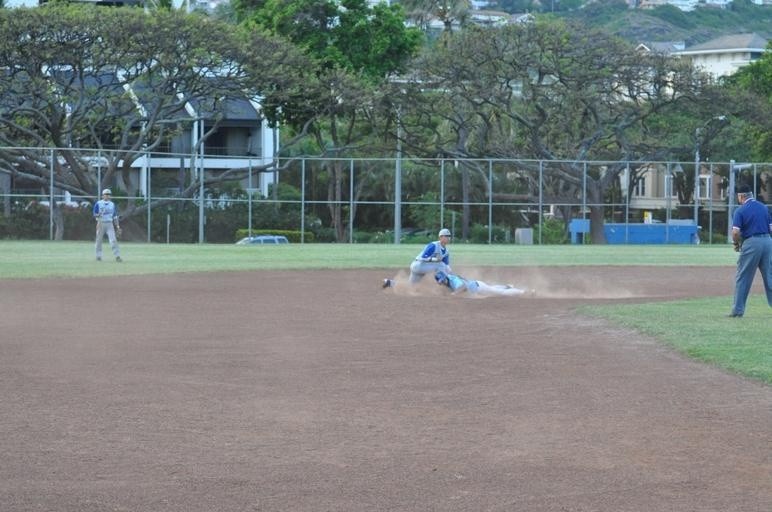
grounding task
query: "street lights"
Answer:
[693,114,727,222]
[197,96,228,244]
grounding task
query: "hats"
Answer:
[102,189,111,194]
[438,228,451,237]
[435,271,446,283]
[736,184,750,193]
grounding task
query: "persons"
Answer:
[726,182,772,317]
[382,229,453,294]
[435,272,536,296]
[93,187,124,263]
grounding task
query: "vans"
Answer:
[235,235,289,244]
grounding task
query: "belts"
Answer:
[749,234,771,238]
